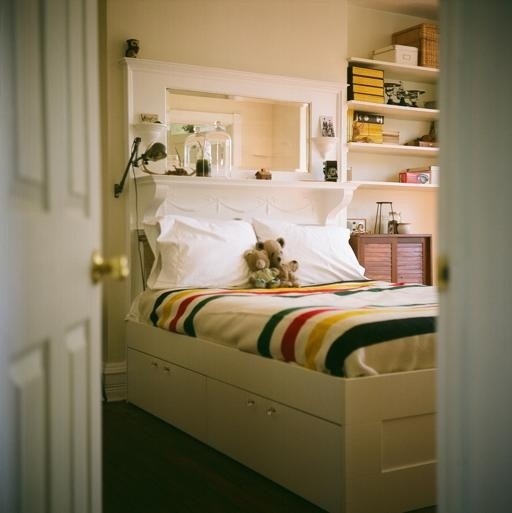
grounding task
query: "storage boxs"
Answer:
[373,45,418,66]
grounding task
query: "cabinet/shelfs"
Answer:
[347,57,441,205]
[349,233,433,286]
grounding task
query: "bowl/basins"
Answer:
[140,113,158,122]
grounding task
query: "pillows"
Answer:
[252,217,374,287]
[143,216,257,290]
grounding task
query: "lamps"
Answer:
[113,136,168,198]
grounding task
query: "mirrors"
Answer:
[165,87,312,173]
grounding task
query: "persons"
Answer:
[322,117,335,136]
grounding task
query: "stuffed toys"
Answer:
[255,236,301,287]
[244,248,281,288]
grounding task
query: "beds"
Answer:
[121,59,440,513]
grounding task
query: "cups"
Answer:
[347,167,352,181]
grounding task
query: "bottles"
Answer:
[183,126,211,176]
[202,120,233,178]
[388,211,412,235]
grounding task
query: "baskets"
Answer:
[393,23,438,67]
